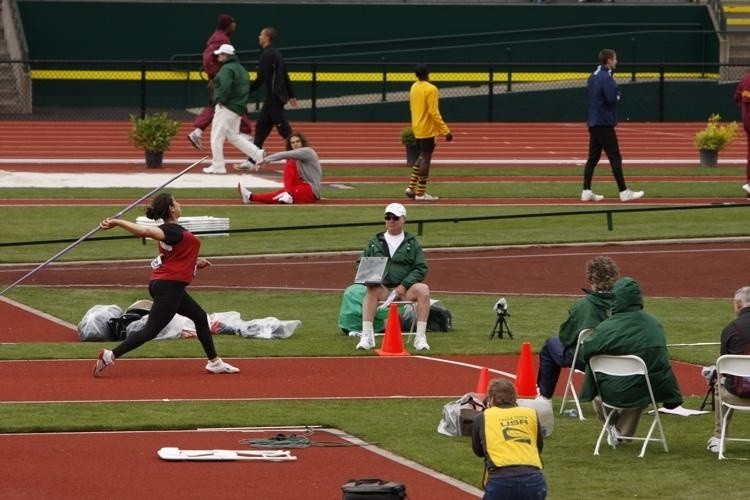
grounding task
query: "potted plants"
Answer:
[128,111,181,168]
[694,112,739,168]
[401,127,418,167]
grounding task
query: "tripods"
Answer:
[490,318,514,339]
[700,388,715,410]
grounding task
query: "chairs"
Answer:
[589,355,669,458]
[559,328,594,421]
[366,301,418,348]
[716,354,750,460]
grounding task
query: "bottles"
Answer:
[564,407,578,418]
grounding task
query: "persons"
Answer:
[733,71,749,197]
[573,274,685,451]
[201,43,265,177]
[705,285,750,454]
[353,202,434,353]
[231,25,297,172]
[91,190,241,378]
[534,255,623,396]
[581,48,650,205]
[469,377,549,498]
[237,131,322,204]
[187,14,254,153]
[404,65,454,202]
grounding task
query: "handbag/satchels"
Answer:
[443,395,486,435]
[340,478,406,500]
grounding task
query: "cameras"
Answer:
[497,303,504,309]
[703,369,718,381]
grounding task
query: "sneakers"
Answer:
[742,183,750,193]
[272,192,293,203]
[413,336,430,351]
[415,193,439,200]
[92,349,114,378]
[355,333,375,350]
[238,181,252,204]
[232,162,249,171]
[706,437,726,452]
[619,189,644,202]
[205,357,240,374]
[253,149,266,172]
[202,166,227,174]
[606,425,621,446]
[187,132,202,149]
[581,189,604,202]
[405,188,414,198]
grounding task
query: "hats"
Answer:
[213,43,236,54]
[385,203,406,216]
[217,14,234,29]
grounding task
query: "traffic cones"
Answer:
[374,302,413,359]
[470,367,492,401]
[509,340,541,403]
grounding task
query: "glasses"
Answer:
[384,214,399,221]
